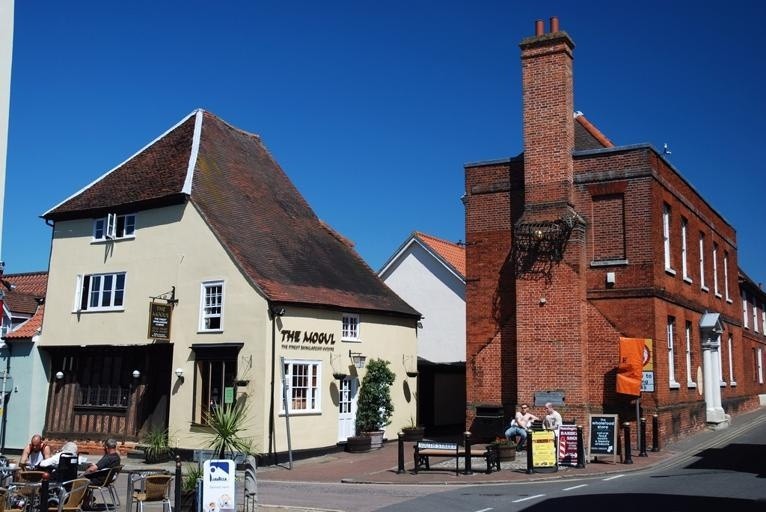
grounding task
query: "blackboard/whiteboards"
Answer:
[588,414,618,456]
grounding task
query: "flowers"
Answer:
[491,437,514,445]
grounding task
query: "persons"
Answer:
[505,403,541,452]
[14,434,52,488]
[542,402,563,447]
[76,438,121,509]
[40,441,87,470]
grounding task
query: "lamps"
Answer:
[174,368,185,383]
[132,369,141,379]
[55,371,64,380]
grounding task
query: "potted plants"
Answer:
[179,461,205,511]
[402,417,426,442]
[138,422,175,464]
[356,357,395,451]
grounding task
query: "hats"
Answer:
[105,438,117,448]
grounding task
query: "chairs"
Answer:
[0,453,172,512]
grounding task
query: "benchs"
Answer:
[413,441,501,474]
[506,417,576,443]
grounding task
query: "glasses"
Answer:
[521,407,527,409]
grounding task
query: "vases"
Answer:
[347,437,371,453]
